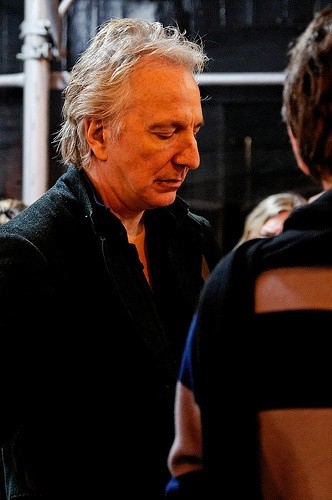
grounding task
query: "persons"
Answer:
[0,16,227,500]
[240,193,306,246]
[166,5,332,500]
